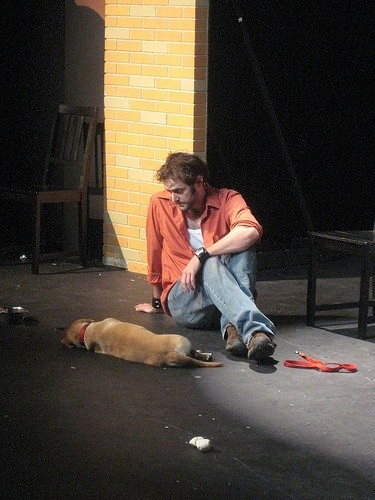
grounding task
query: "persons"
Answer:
[133,153,277,361]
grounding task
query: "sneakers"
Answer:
[225,326,247,355]
[247,332,274,359]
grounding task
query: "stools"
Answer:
[305,229,375,338]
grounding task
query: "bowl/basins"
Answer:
[0,312,24,324]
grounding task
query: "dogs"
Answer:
[60,319,225,369]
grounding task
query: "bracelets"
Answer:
[151,295,162,309]
[194,247,210,263]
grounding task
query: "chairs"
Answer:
[0,104,99,273]
